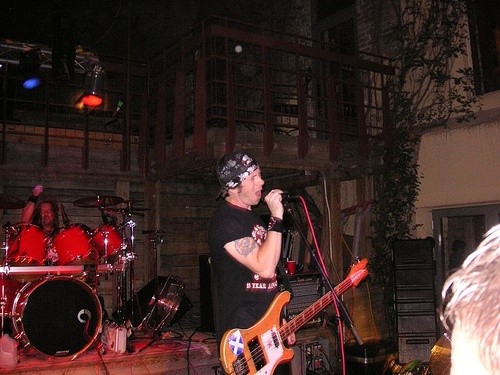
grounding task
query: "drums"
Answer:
[50,223,95,267]
[1,222,51,267]
[0,269,25,316]
[133,275,185,335]
[10,274,104,364]
[89,222,128,261]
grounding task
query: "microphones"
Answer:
[142,230,167,234]
[261,192,300,202]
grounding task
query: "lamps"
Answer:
[81,62,106,107]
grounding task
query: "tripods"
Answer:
[138,234,199,352]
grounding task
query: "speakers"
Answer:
[113,276,193,333]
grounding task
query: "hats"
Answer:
[215,151,258,202]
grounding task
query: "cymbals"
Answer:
[122,198,145,205]
[73,191,125,208]
[121,206,152,213]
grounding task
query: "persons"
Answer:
[209,151,296,375]
[440,224,500,375]
[21,185,64,238]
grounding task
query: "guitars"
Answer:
[219,257,369,375]
